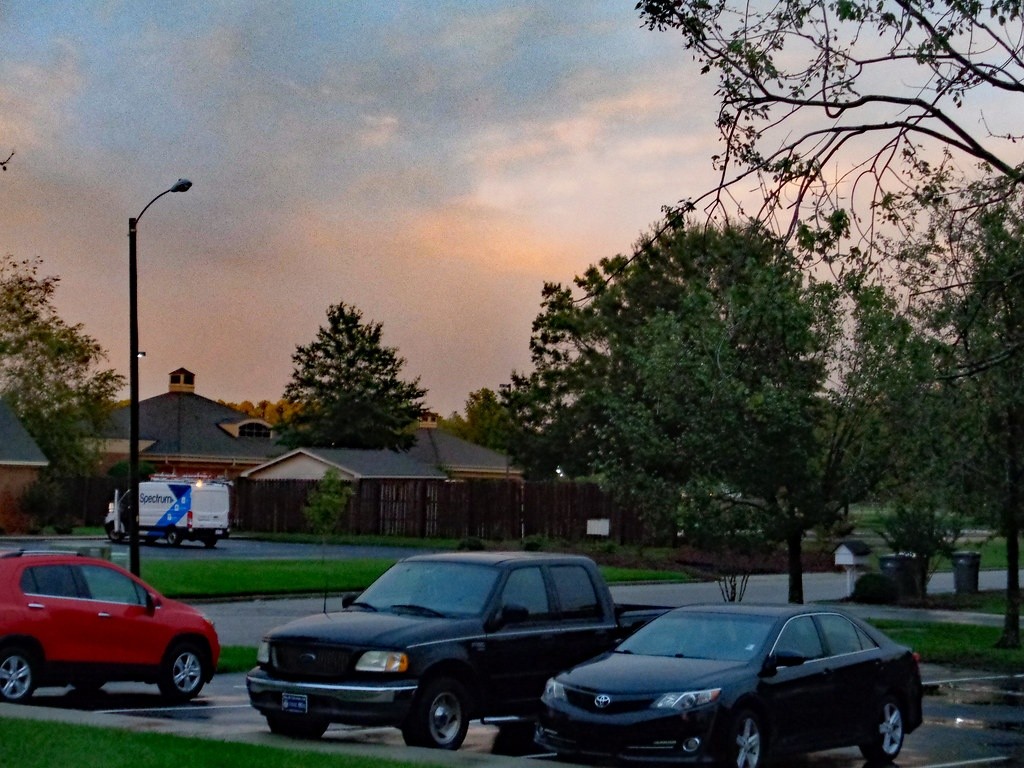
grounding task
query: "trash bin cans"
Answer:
[77,546,111,561]
[879,554,924,599]
[954,552,979,593]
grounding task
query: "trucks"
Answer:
[104,472,233,547]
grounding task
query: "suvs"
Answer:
[0,549,220,703]
[247,551,623,750]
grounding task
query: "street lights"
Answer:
[129,178,193,582]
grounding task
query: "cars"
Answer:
[532,602,923,768]
